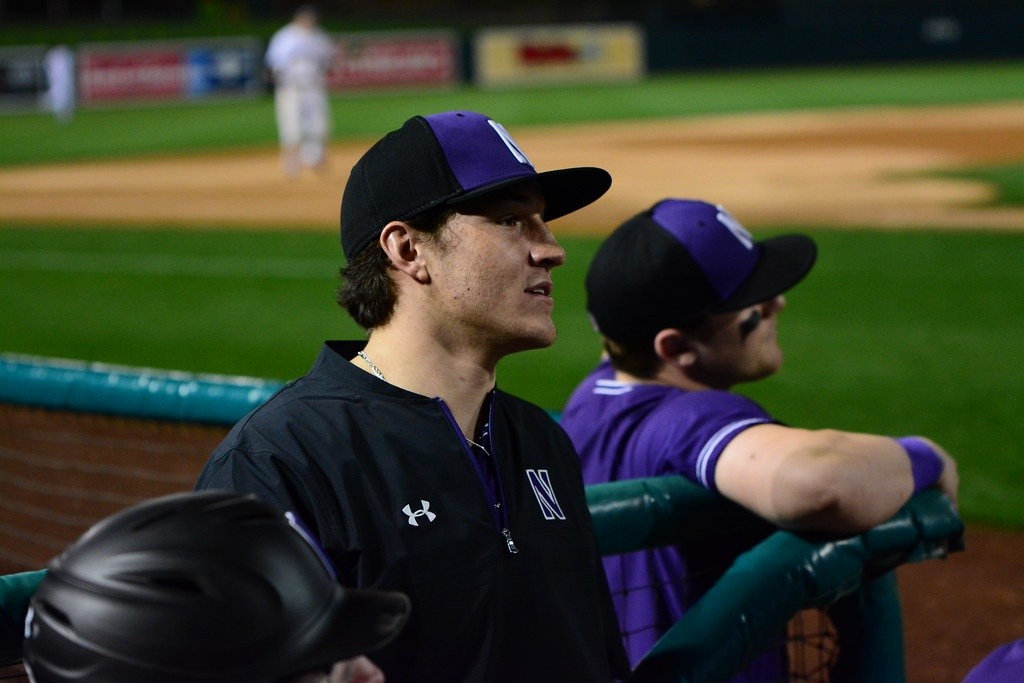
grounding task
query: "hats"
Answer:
[585,198,818,340]
[340,109,613,255]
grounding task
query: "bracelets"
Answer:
[899,436,945,493]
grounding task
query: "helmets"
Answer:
[21,490,411,683]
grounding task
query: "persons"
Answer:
[264,7,338,165]
[44,42,76,113]
[561,199,960,683]
[23,492,412,683]
[190,113,633,683]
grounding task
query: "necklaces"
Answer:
[357,352,490,457]
